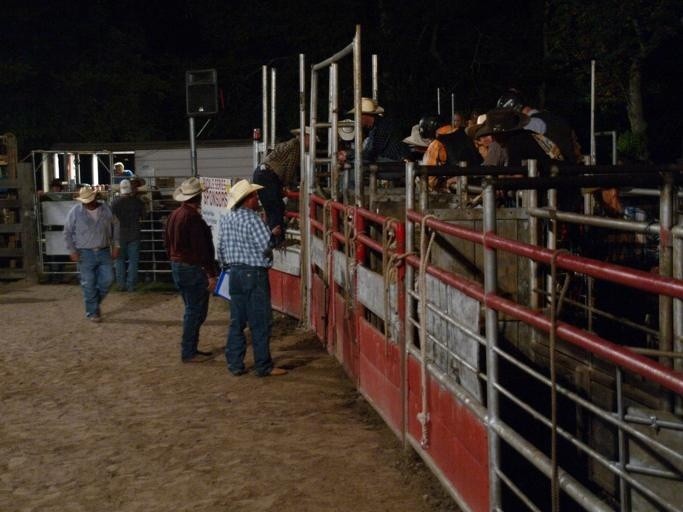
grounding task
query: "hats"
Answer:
[173,177,210,202]
[578,166,633,193]
[346,98,385,115]
[291,126,320,142]
[226,179,265,210]
[402,124,430,148]
[130,174,146,186]
[73,186,99,204]
[120,179,133,195]
[475,108,530,138]
[338,119,356,141]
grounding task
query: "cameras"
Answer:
[270,221,288,231]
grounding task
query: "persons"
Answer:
[164,177,218,361]
[337,97,582,193]
[43,162,148,324]
[219,179,288,376]
[253,126,320,245]
[596,168,661,270]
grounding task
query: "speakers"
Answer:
[185,68,219,117]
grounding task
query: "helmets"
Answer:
[496,88,527,111]
[419,112,447,145]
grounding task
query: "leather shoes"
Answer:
[268,368,287,375]
[183,350,213,362]
